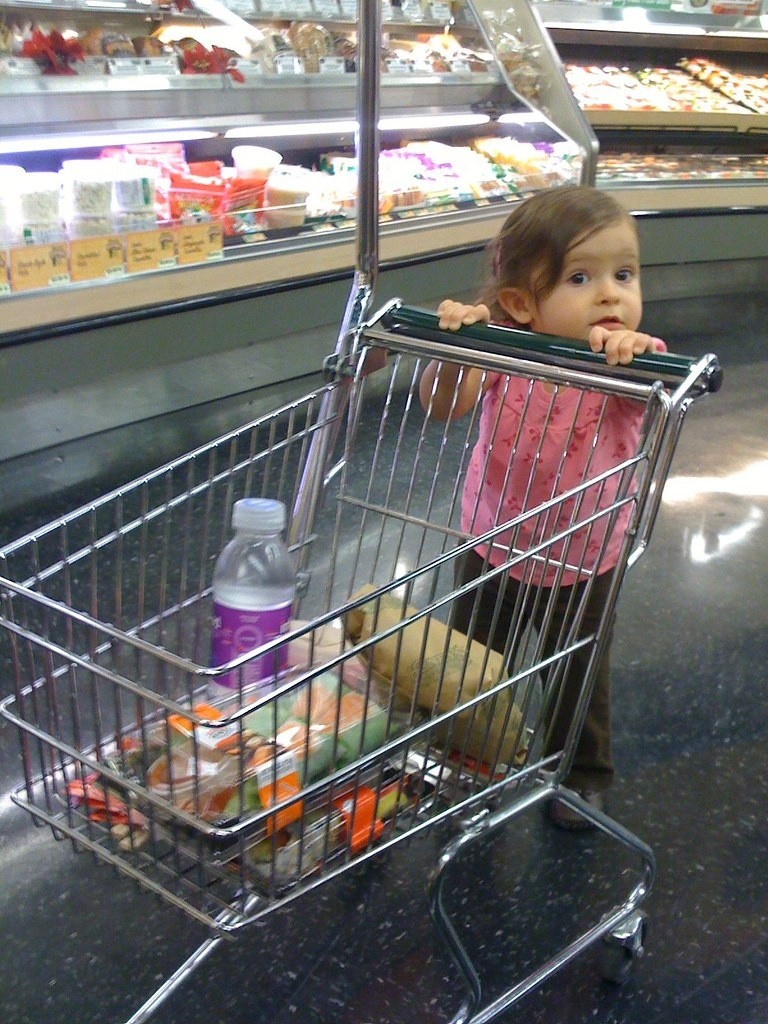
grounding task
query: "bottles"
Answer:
[206,498,298,718]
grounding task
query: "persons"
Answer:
[417,184,666,825]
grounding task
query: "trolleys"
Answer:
[1,297,726,1024]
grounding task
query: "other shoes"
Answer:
[550,789,605,831]
